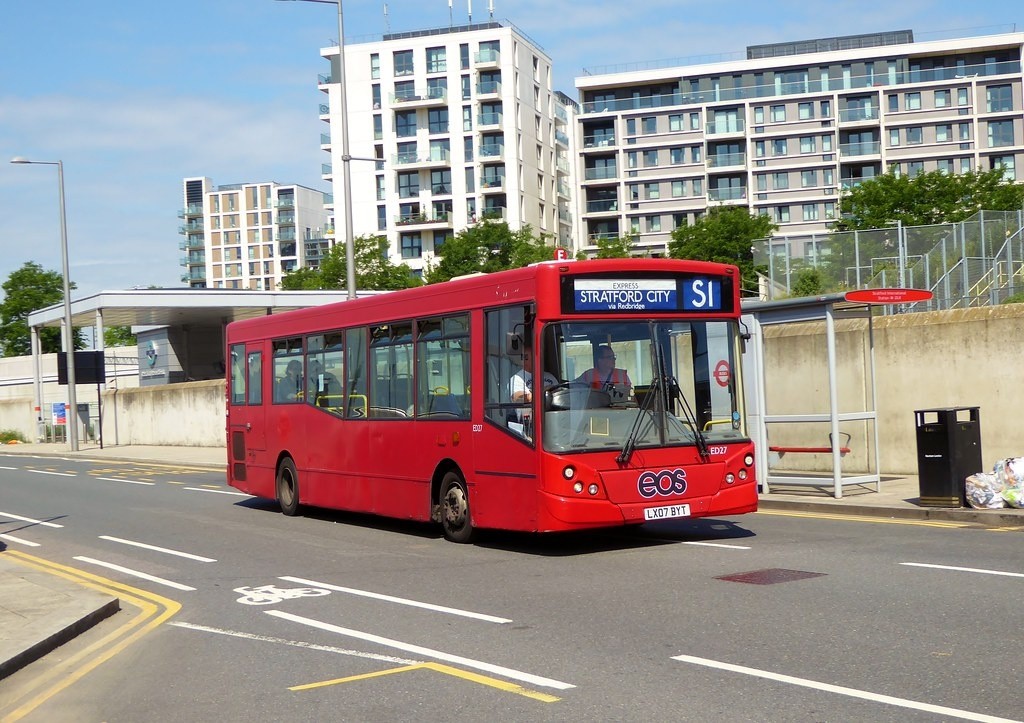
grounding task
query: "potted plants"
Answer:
[401,154,431,164]
[399,94,421,102]
[395,211,448,226]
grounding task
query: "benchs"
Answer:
[768,431,851,470]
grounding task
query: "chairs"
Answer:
[506,409,533,436]
[274,376,471,419]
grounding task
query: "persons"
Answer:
[507,342,561,435]
[572,345,631,404]
[280,360,343,407]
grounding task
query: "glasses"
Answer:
[601,355,616,360]
[521,353,529,361]
[291,368,302,371]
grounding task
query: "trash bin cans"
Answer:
[911,405,984,509]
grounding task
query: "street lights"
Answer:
[10,156,81,451]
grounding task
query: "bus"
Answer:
[225,258,758,544]
